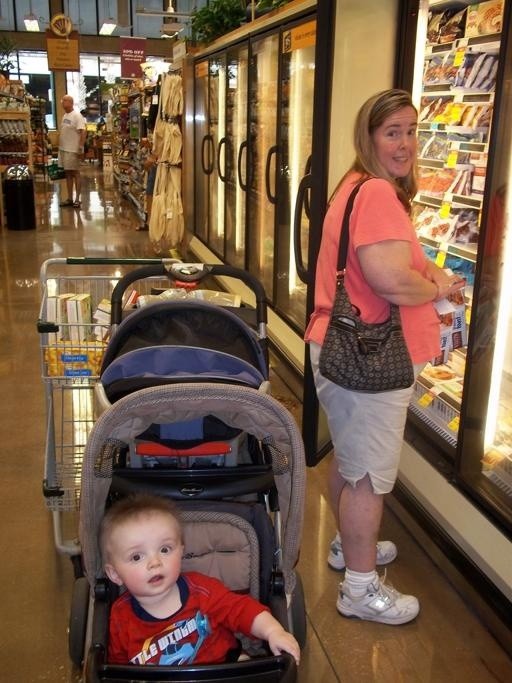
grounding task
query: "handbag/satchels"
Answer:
[317,278,415,393]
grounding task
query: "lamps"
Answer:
[134,7,197,19]
[98,0,118,35]
[21,0,41,31]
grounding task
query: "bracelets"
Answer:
[431,279,441,303]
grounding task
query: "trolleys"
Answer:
[38,255,269,567]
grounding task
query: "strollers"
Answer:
[68,265,300,682]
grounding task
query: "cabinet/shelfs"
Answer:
[109,79,152,230]
[0,73,53,183]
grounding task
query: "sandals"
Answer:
[59,198,73,206]
[73,201,80,208]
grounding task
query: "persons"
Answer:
[98,490,302,668]
[303,87,468,625]
[58,95,87,208]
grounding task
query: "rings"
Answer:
[447,284,451,288]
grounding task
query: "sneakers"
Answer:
[336,577,420,626]
[327,530,398,571]
